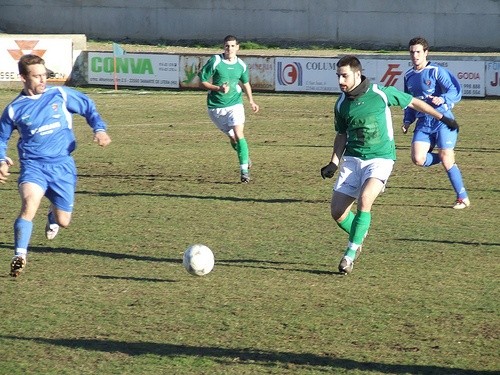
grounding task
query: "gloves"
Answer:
[438,115,460,134]
[319,161,338,180]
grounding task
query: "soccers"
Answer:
[182,242,213,277]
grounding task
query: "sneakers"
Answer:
[351,228,368,260]
[240,169,250,183]
[337,255,355,277]
[43,204,61,240]
[452,197,471,210]
[9,253,27,278]
[246,158,253,169]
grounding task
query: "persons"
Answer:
[320,55,459,274]
[1,55,112,278]
[402,37,471,209]
[199,35,258,185]
